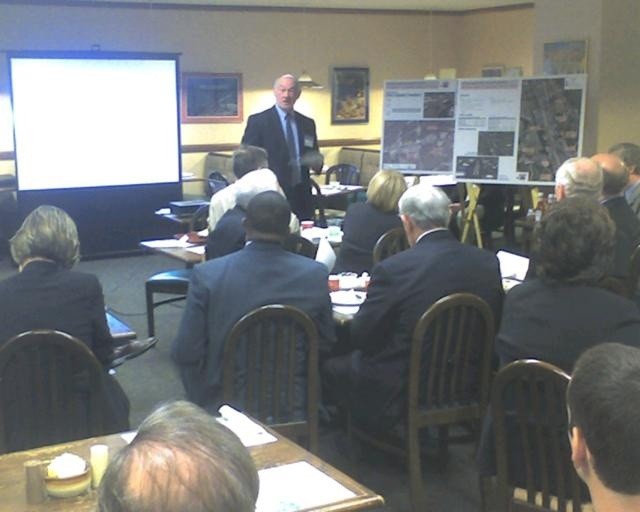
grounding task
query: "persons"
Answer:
[241,75,323,227]
[564,352,640,511]
[472,141,639,504]
[324,183,504,428]
[97,398,259,512]
[204,144,412,275]
[170,193,341,427]
[1,206,160,455]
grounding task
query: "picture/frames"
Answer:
[540,38,589,75]
[182,72,244,123]
[479,65,504,78]
[330,67,368,124]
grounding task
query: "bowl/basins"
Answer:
[44,462,93,497]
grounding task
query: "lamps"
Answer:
[424,8,438,80]
[295,9,322,90]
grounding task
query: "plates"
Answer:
[329,290,368,305]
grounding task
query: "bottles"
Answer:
[91,444,109,485]
[24,459,47,502]
[536,193,554,218]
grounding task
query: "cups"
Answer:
[302,221,315,228]
[328,275,339,288]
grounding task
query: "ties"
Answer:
[286,116,298,184]
